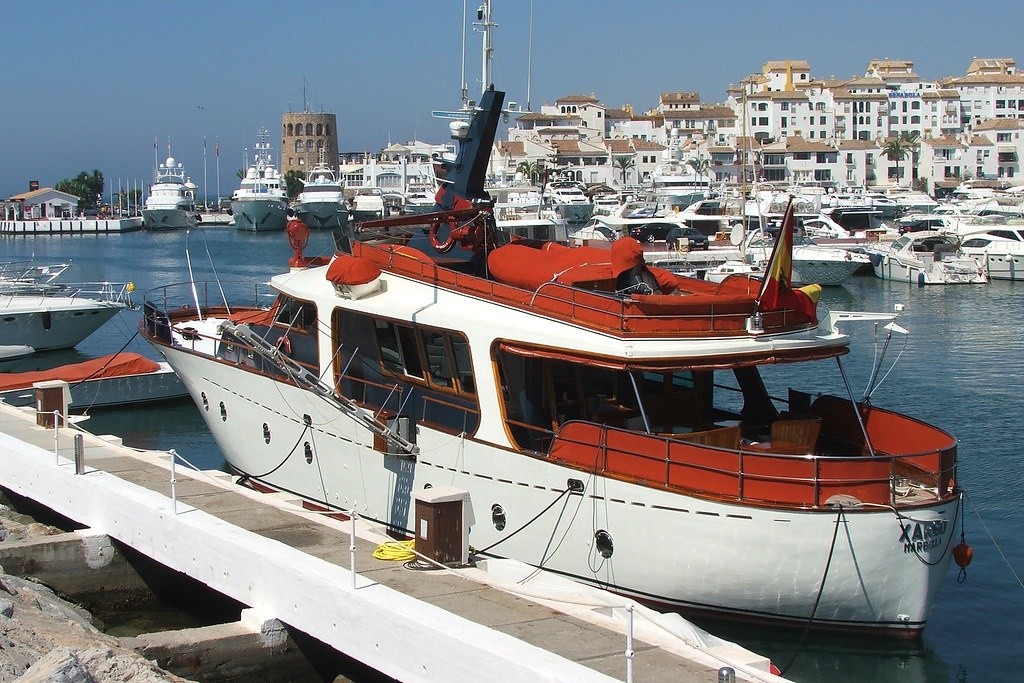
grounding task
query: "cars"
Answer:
[122,208,132,216]
[546,189,590,214]
[630,222,689,243]
[898,219,950,236]
[584,185,617,196]
[665,228,709,251]
[83,207,101,216]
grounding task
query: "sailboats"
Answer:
[707,85,766,283]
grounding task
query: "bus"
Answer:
[595,200,620,210]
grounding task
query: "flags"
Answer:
[152,140,219,157]
[758,203,823,324]
[147,185,152,196]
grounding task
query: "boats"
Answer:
[348,187,389,225]
[867,231,988,286]
[753,243,871,286]
[958,226,1024,281]
[226,126,294,231]
[140,136,202,231]
[136,0,973,642]
[0,261,140,359]
[893,205,980,226]
[292,147,350,231]
[827,192,941,219]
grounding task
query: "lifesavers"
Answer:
[426,212,461,257]
[770,201,814,212]
